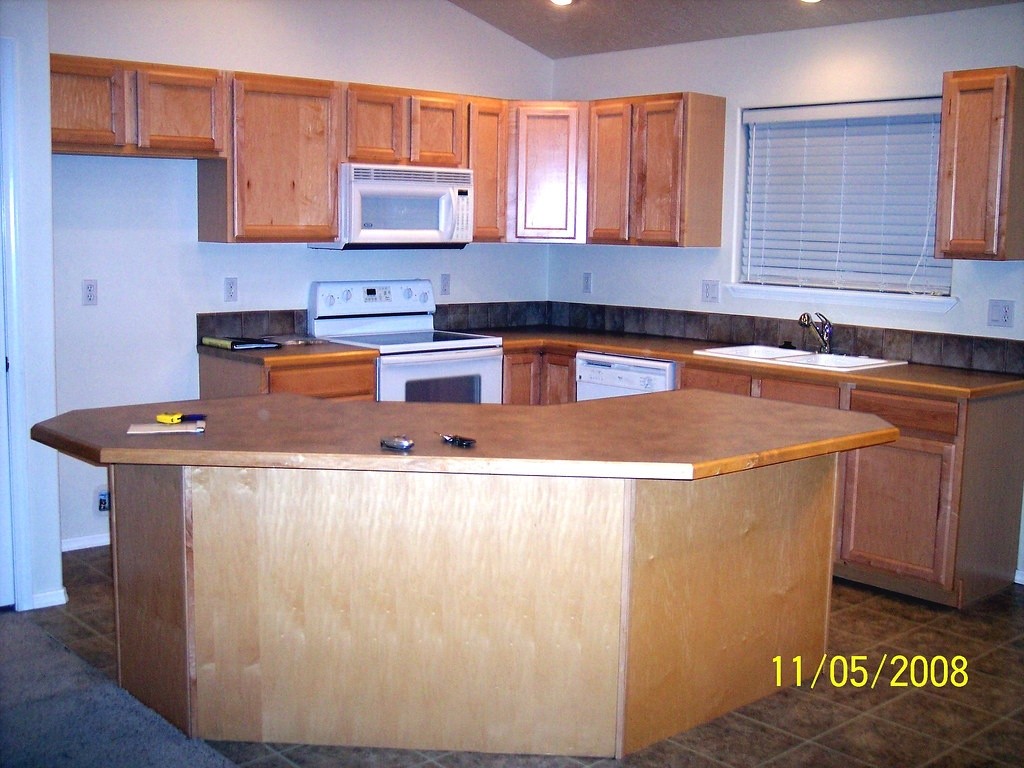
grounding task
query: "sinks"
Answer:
[767,353,909,372]
[693,345,820,363]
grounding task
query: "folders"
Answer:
[201,335,282,350]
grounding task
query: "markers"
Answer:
[181,414,206,420]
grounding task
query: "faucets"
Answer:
[798,311,834,354]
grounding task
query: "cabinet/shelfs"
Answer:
[839,388,1024,609]
[199,351,377,403]
[586,92,723,247]
[673,362,840,409]
[503,347,576,407]
[508,97,587,244]
[464,94,510,244]
[50,53,227,159]
[198,67,342,244]
[934,66,1024,261]
[342,81,466,170]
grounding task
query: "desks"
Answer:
[30,388,900,760]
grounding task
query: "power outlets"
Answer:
[988,299,1014,327]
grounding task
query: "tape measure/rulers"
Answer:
[156,412,183,424]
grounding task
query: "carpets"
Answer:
[0,607,245,768]
